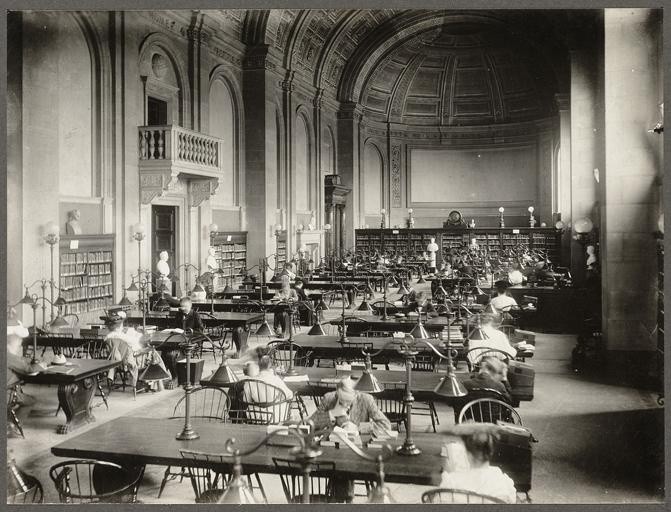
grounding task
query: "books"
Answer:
[357,234,434,256]
[266,424,301,447]
[58,252,114,317]
[49,365,74,375]
[441,325,464,350]
[160,328,184,334]
[318,425,363,449]
[277,241,286,272]
[442,233,561,261]
[213,244,246,289]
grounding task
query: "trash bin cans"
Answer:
[177,358,204,387]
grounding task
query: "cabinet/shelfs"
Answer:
[59,234,114,315]
[275,230,287,273]
[214,231,249,292]
[354,227,561,268]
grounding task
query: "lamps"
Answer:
[209,224,217,246]
[528,206,536,228]
[297,223,304,232]
[44,222,60,245]
[554,220,566,245]
[273,223,281,239]
[406,208,414,229]
[324,223,330,232]
[131,223,147,242]
[498,206,505,227]
[574,216,594,268]
[380,209,386,227]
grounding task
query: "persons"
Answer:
[175,296,203,331]
[307,378,391,504]
[293,280,306,301]
[274,282,298,302]
[104,310,144,367]
[586,245,596,265]
[508,262,522,284]
[7,333,48,439]
[447,356,515,424]
[432,430,517,504]
[155,250,170,277]
[243,354,293,423]
[403,291,439,317]
[427,238,438,251]
[490,280,518,315]
[66,209,82,235]
